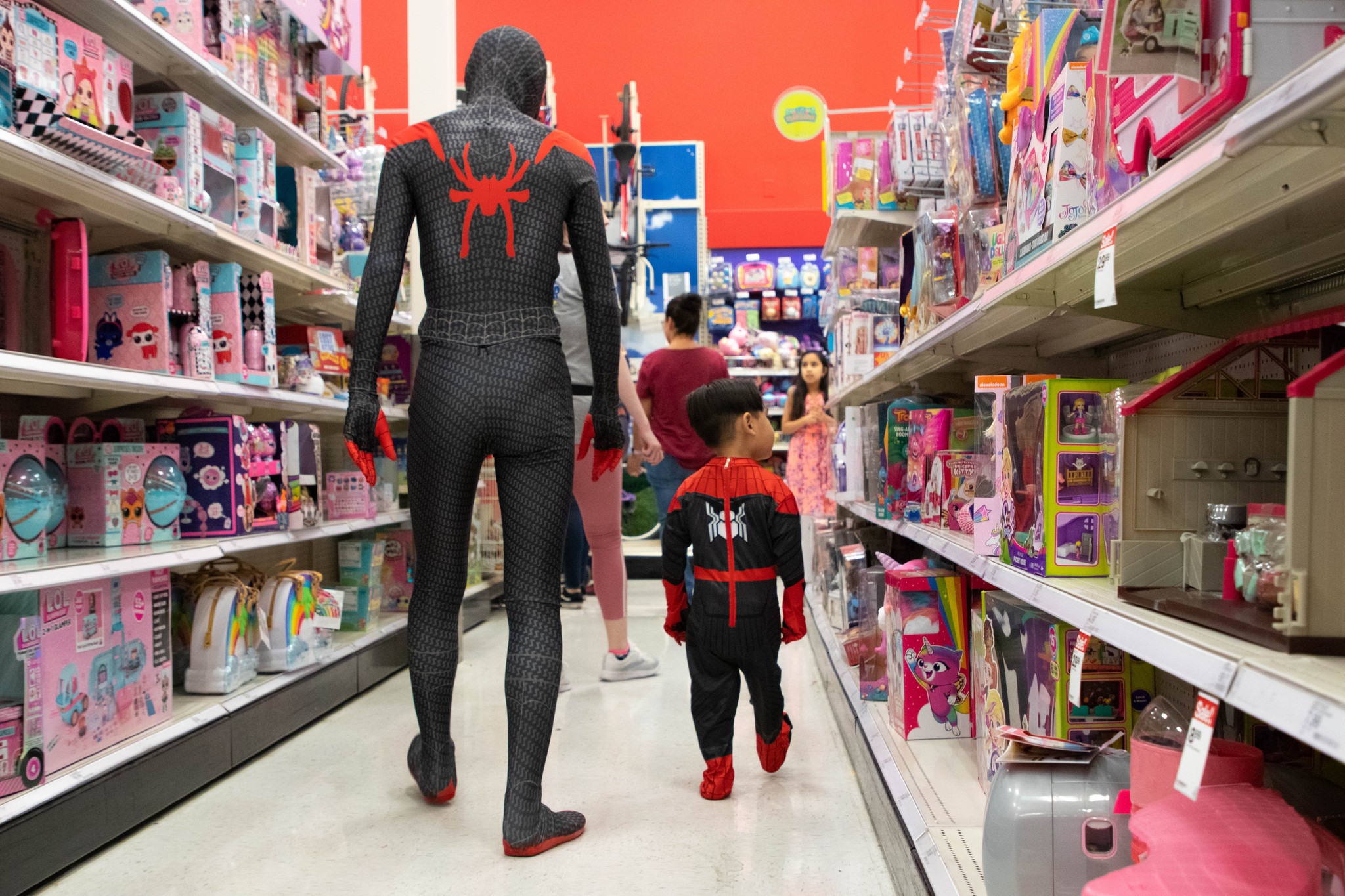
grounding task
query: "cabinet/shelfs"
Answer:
[267,287,503,691]
[803,44,1345,896]
[0,0,222,877]
[128,69,359,775]
[729,364,798,486]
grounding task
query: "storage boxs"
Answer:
[2,0,1345,896]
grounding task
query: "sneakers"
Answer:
[598,640,660,682]
[560,588,584,610]
[557,661,572,692]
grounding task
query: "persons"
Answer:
[557,494,602,610]
[661,378,808,799]
[341,24,628,857]
[781,349,838,587]
[983,399,997,461]
[628,292,733,613]
[549,189,671,682]
[1065,398,1093,435]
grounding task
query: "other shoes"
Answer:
[502,803,587,856]
[406,732,458,807]
[754,713,793,773]
[700,753,735,801]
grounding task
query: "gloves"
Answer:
[781,578,808,645]
[577,413,626,482]
[343,405,397,486]
[662,578,689,647]
